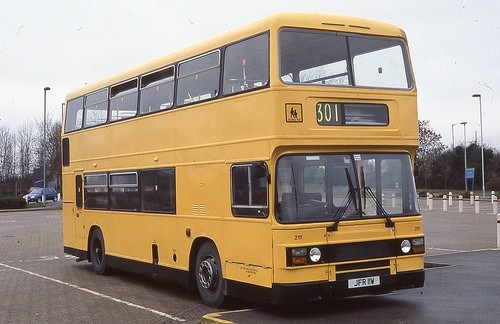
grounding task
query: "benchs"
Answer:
[86,79,264,128]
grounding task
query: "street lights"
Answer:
[42,86,51,207]
[452,123,457,148]
[471,94,486,198]
[460,122,469,193]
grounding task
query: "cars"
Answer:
[23,187,59,204]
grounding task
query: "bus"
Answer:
[62,11,427,308]
